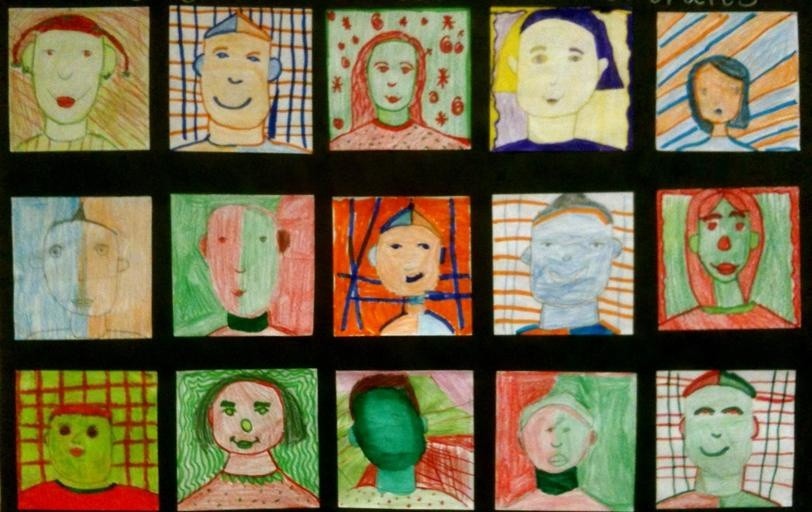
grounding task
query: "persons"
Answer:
[19,373,782,512]
[24,190,799,340]
[12,9,760,155]
